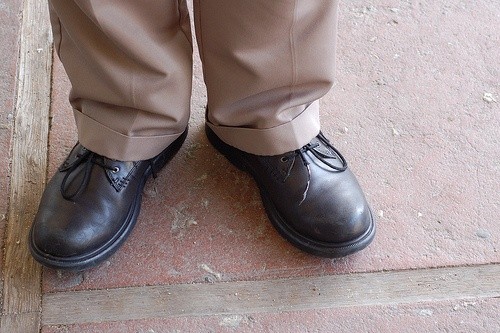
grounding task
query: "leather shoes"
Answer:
[204,124,375,258]
[29,124,193,268]
